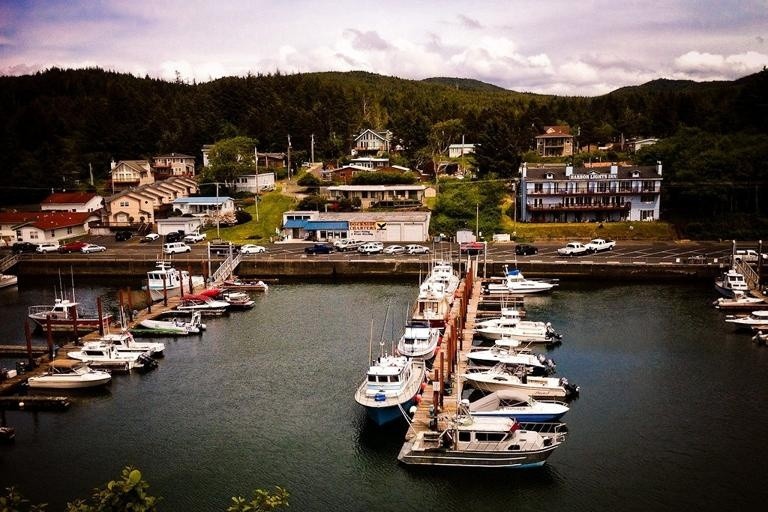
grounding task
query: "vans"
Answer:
[163,242,191,254]
[35,242,60,253]
[12,242,39,252]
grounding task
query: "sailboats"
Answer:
[146,228,205,301]
[27,265,113,332]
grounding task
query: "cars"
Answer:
[61,240,89,253]
[80,243,107,254]
[240,244,266,255]
[167,232,181,242]
[144,233,160,241]
[305,243,334,255]
[115,230,132,241]
[184,232,207,243]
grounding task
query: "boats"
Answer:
[139,311,206,334]
[714,267,768,344]
[1,273,18,289]
[201,285,255,310]
[355,304,426,408]
[475,296,564,343]
[99,334,166,353]
[457,389,570,421]
[410,269,451,327]
[396,302,440,360]
[418,242,460,306]
[489,252,561,284]
[66,340,156,376]
[475,293,553,329]
[27,359,110,390]
[176,293,231,316]
[223,277,268,291]
[465,323,556,372]
[463,361,578,398]
[402,394,569,468]
[488,264,560,294]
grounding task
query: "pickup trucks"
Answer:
[334,238,365,251]
[559,241,591,256]
[587,236,616,254]
[732,249,767,262]
